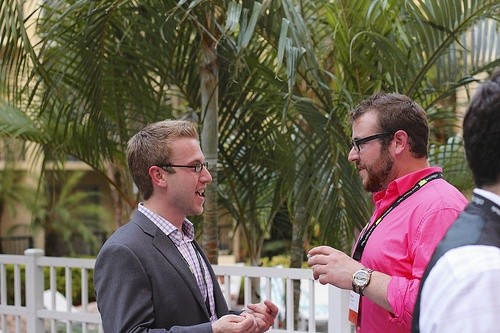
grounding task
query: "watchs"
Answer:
[351,268,373,296]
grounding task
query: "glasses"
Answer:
[349,131,394,152]
[157,162,208,173]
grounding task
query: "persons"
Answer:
[91,119,280,333]
[307,91,469,333]
[410,73,500,333]
